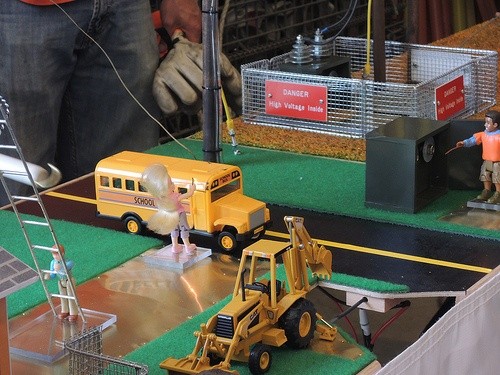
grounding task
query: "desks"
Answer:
[0,206,383,375]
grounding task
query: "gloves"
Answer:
[153,28,256,113]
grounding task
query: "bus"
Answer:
[95,150,271,254]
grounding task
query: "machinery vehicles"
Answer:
[159,214,338,375]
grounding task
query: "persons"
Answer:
[456,110,500,204]
[135,164,200,256]
[44,244,78,323]
[1,0,203,206]
